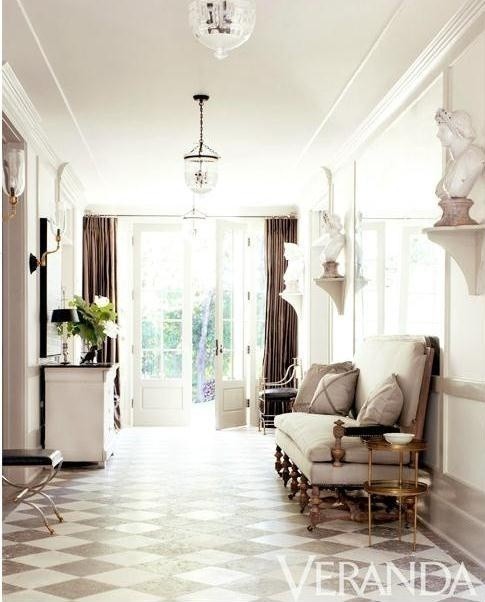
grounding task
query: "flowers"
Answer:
[56,293,120,350]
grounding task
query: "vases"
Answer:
[97,349,103,363]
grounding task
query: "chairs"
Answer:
[257,362,303,435]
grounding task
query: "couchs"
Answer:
[274,333,435,533]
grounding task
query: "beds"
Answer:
[363,434,429,554]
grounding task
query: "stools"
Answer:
[2,448,64,535]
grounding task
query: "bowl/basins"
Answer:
[382,433,415,445]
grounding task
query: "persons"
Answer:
[321,213,345,262]
[283,242,304,282]
[435,108,485,198]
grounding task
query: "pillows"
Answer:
[292,361,352,412]
[357,373,403,427]
[308,368,360,417]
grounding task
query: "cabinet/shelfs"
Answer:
[44,363,120,470]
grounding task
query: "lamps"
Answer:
[2,142,25,227]
[188,0,256,60]
[29,211,66,276]
[183,94,222,193]
[51,308,80,364]
[181,195,206,237]
